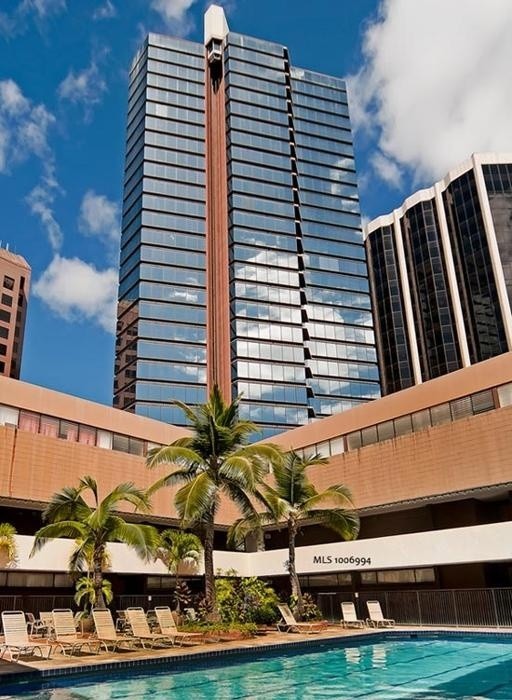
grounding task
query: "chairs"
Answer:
[366,600,395,629]
[0,606,203,662]
[276,603,323,634]
[341,602,365,628]
